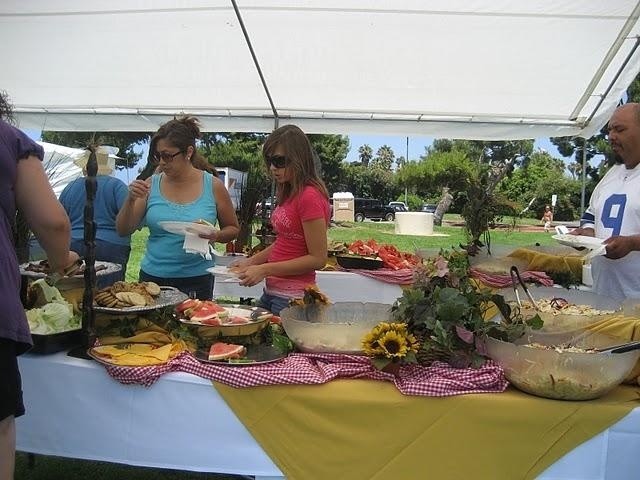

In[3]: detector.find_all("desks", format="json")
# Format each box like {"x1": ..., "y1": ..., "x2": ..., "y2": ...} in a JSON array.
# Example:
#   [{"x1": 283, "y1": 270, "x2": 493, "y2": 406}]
[
  {"x1": 203, "y1": 267, "x2": 590, "y2": 322},
  {"x1": 15, "y1": 340, "x2": 640, "y2": 480}
]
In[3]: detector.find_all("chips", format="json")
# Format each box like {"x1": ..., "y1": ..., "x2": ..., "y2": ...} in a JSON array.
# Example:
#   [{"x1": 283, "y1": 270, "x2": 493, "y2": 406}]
[{"x1": 93, "y1": 343, "x2": 182, "y2": 365}]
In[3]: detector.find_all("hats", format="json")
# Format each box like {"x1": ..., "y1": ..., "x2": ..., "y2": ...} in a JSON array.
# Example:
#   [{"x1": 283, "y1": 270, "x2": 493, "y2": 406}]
[{"x1": 72, "y1": 146, "x2": 114, "y2": 176}]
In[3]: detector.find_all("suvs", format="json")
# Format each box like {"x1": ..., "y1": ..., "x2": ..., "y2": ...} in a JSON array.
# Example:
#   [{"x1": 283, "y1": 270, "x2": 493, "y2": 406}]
[
  {"x1": 354, "y1": 197, "x2": 396, "y2": 224},
  {"x1": 256, "y1": 196, "x2": 278, "y2": 217}
]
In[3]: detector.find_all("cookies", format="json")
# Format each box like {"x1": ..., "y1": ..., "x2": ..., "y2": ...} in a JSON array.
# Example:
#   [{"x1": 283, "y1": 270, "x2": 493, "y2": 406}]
[{"x1": 94, "y1": 280, "x2": 161, "y2": 308}]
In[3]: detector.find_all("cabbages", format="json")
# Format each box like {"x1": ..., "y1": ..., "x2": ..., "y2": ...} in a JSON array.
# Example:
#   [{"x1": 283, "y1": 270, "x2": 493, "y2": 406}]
[{"x1": 25, "y1": 279, "x2": 80, "y2": 334}]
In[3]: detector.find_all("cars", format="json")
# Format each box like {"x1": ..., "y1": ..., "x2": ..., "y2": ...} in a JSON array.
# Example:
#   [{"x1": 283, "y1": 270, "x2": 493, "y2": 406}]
[
  {"x1": 329, "y1": 197, "x2": 334, "y2": 217},
  {"x1": 390, "y1": 201, "x2": 437, "y2": 214}
]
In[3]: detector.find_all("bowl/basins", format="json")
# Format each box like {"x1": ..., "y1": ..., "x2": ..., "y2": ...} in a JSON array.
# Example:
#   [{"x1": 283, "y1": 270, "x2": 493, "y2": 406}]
[
  {"x1": 482, "y1": 326, "x2": 640, "y2": 401},
  {"x1": 414, "y1": 248, "x2": 448, "y2": 259},
  {"x1": 23, "y1": 287, "x2": 275, "y2": 351},
  {"x1": 336, "y1": 254, "x2": 383, "y2": 270},
  {"x1": 501, "y1": 301, "x2": 624, "y2": 326},
  {"x1": 280, "y1": 300, "x2": 397, "y2": 355}
]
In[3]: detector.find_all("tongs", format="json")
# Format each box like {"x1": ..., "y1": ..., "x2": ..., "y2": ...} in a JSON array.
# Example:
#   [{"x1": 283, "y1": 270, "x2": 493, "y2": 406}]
[
  {"x1": 585, "y1": 338, "x2": 639, "y2": 353},
  {"x1": 509, "y1": 263, "x2": 540, "y2": 309}
]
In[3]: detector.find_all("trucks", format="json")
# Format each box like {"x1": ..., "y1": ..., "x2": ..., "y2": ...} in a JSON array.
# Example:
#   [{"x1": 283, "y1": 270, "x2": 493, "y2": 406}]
[{"x1": 213, "y1": 165, "x2": 248, "y2": 213}]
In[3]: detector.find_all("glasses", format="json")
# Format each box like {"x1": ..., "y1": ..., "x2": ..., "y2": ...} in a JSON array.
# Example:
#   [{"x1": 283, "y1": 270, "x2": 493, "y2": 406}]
[
  {"x1": 151, "y1": 148, "x2": 185, "y2": 164},
  {"x1": 263, "y1": 154, "x2": 297, "y2": 168}
]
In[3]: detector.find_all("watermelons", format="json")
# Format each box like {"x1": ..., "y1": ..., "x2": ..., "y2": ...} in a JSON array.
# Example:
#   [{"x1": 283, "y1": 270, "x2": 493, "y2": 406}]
[
  {"x1": 177, "y1": 298, "x2": 248, "y2": 326},
  {"x1": 271, "y1": 316, "x2": 282, "y2": 325},
  {"x1": 209, "y1": 341, "x2": 247, "y2": 361}
]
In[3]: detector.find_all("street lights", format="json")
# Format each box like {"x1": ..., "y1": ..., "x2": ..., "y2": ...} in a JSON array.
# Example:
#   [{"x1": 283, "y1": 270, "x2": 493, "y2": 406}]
[{"x1": 106, "y1": 153, "x2": 131, "y2": 185}]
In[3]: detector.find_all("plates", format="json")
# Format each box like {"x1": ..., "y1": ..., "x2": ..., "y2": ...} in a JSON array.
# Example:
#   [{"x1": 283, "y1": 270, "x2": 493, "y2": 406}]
[
  {"x1": 204, "y1": 266, "x2": 245, "y2": 276},
  {"x1": 551, "y1": 232, "x2": 603, "y2": 248},
  {"x1": 157, "y1": 221, "x2": 215, "y2": 238}
]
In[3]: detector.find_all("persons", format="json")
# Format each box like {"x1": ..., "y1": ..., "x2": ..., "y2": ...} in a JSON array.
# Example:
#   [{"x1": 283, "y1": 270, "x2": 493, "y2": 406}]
[
  {"x1": 566, "y1": 102, "x2": 640, "y2": 303},
  {"x1": 59, "y1": 148, "x2": 142, "y2": 288},
  {"x1": 541, "y1": 205, "x2": 553, "y2": 232},
  {"x1": 1, "y1": 92, "x2": 82, "y2": 479},
  {"x1": 224, "y1": 123, "x2": 330, "y2": 320},
  {"x1": 115, "y1": 114, "x2": 240, "y2": 300}
]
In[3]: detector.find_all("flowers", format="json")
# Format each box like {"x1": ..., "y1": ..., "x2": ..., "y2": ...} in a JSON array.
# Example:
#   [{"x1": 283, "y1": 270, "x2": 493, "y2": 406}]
[{"x1": 358, "y1": 252, "x2": 493, "y2": 364}]
